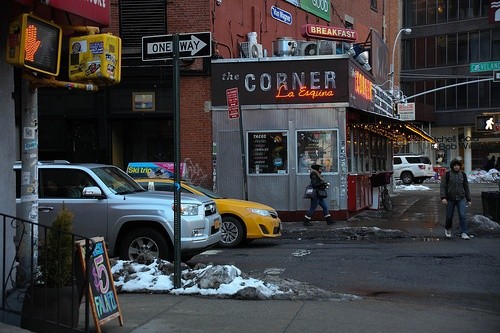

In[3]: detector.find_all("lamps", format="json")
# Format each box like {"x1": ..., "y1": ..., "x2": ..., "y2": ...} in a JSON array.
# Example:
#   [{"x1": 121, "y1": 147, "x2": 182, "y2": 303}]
[
  {"x1": 357, "y1": 50, "x2": 371, "y2": 71},
  {"x1": 378, "y1": 80, "x2": 407, "y2": 107}
]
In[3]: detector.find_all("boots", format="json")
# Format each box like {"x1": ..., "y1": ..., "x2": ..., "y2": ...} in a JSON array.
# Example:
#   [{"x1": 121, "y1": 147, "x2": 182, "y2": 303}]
[
  {"x1": 324, "y1": 214, "x2": 336, "y2": 225},
  {"x1": 303, "y1": 215, "x2": 313, "y2": 226}
]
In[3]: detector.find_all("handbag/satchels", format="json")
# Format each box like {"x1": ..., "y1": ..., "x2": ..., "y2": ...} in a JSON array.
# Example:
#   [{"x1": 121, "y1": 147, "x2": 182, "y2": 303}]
[
  {"x1": 303, "y1": 185, "x2": 314, "y2": 199},
  {"x1": 316, "y1": 190, "x2": 327, "y2": 198}
]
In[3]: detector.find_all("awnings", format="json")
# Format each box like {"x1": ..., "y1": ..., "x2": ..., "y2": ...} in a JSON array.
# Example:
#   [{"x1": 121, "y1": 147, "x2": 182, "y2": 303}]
[{"x1": 350, "y1": 107, "x2": 436, "y2": 145}]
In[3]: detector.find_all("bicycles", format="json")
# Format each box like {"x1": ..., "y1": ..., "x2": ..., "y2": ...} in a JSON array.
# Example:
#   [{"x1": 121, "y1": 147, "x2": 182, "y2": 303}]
[{"x1": 380, "y1": 171, "x2": 394, "y2": 211}]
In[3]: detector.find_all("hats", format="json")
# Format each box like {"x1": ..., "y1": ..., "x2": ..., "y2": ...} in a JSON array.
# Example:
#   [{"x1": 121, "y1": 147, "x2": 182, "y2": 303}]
[{"x1": 311, "y1": 164, "x2": 323, "y2": 170}]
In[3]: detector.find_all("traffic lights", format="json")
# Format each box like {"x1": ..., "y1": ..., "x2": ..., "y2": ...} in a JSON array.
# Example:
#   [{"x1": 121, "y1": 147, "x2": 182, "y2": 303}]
[
  {"x1": 6, "y1": 12, "x2": 62, "y2": 77},
  {"x1": 475, "y1": 114, "x2": 496, "y2": 132},
  {"x1": 432, "y1": 143, "x2": 439, "y2": 148},
  {"x1": 493, "y1": 69, "x2": 500, "y2": 82}
]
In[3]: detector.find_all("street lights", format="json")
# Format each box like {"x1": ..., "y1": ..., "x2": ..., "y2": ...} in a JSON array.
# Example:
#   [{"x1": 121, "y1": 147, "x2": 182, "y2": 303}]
[{"x1": 392, "y1": 29, "x2": 411, "y2": 92}]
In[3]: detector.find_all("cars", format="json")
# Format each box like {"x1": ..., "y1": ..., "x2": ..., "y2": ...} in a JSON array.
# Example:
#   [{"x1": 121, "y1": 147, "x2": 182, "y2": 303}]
[
  {"x1": 432, "y1": 162, "x2": 446, "y2": 178},
  {"x1": 112, "y1": 162, "x2": 278, "y2": 247}
]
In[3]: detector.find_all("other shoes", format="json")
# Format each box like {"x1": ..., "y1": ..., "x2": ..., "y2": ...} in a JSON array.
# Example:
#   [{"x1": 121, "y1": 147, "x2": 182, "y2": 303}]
[
  {"x1": 462, "y1": 232, "x2": 469, "y2": 240},
  {"x1": 444, "y1": 228, "x2": 451, "y2": 238}
]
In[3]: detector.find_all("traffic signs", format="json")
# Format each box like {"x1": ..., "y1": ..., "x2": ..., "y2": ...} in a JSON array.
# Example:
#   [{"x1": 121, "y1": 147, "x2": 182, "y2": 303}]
[{"x1": 141, "y1": 31, "x2": 212, "y2": 61}]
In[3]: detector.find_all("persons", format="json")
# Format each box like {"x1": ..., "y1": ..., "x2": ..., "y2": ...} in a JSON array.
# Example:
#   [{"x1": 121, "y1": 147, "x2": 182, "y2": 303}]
[
  {"x1": 302, "y1": 162, "x2": 336, "y2": 227},
  {"x1": 440, "y1": 158, "x2": 472, "y2": 240}
]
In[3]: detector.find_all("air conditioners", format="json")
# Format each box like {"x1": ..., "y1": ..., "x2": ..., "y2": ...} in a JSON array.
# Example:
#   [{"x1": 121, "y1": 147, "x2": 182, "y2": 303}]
[{"x1": 240, "y1": 42, "x2": 264, "y2": 58}]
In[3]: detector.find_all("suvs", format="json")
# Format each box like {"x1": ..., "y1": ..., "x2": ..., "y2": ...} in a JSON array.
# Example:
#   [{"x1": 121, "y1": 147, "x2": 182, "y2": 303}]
[
  {"x1": 392, "y1": 153, "x2": 436, "y2": 184},
  {"x1": 12, "y1": 160, "x2": 222, "y2": 264}
]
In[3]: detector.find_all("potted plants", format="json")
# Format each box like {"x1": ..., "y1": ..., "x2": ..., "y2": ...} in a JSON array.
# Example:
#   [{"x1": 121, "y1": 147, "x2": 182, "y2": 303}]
[{"x1": 21, "y1": 200, "x2": 85, "y2": 333}]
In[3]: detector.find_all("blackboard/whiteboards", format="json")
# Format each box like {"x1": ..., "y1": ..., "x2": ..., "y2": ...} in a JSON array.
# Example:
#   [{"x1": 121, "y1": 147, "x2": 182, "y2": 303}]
[{"x1": 76, "y1": 237, "x2": 121, "y2": 325}]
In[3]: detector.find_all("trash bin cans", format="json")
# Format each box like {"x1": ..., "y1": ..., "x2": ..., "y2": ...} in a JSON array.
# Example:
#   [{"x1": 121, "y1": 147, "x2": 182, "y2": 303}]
[{"x1": 482, "y1": 191, "x2": 500, "y2": 225}]
